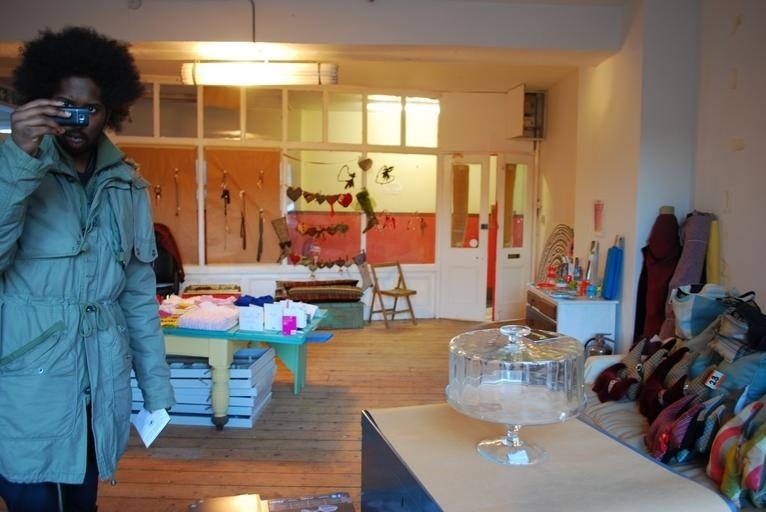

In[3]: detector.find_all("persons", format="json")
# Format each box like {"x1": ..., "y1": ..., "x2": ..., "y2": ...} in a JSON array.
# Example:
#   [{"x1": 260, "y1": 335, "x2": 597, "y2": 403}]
[{"x1": 0, "y1": 24, "x2": 176, "y2": 511}]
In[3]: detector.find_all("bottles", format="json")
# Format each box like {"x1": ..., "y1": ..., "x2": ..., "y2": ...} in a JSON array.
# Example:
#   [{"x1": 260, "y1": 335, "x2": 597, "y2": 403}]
[
  {"x1": 601, "y1": 234, "x2": 623, "y2": 299},
  {"x1": 585, "y1": 240, "x2": 599, "y2": 280},
  {"x1": 573, "y1": 266, "x2": 584, "y2": 281}
]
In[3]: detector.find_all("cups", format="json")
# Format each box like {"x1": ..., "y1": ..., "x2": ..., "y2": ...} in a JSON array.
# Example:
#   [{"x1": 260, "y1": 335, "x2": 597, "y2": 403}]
[
  {"x1": 580, "y1": 281, "x2": 591, "y2": 296},
  {"x1": 586, "y1": 285, "x2": 597, "y2": 298},
  {"x1": 282, "y1": 308, "x2": 296, "y2": 336},
  {"x1": 565, "y1": 275, "x2": 572, "y2": 283}
]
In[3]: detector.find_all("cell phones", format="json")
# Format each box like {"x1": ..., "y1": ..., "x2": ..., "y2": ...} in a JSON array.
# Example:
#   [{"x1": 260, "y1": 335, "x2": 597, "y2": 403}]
[{"x1": 53, "y1": 108, "x2": 90, "y2": 127}]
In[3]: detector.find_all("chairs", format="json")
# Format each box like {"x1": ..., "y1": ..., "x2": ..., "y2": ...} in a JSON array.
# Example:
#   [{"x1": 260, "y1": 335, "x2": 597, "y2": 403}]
[
  {"x1": 366, "y1": 260, "x2": 418, "y2": 328},
  {"x1": 151, "y1": 222, "x2": 184, "y2": 297}
]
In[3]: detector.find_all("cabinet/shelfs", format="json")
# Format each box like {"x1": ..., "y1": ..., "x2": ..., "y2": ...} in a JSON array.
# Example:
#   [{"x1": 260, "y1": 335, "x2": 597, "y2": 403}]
[
  {"x1": 524, "y1": 279, "x2": 620, "y2": 354},
  {"x1": 504, "y1": 85, "x2": 546, "y2": 142},
  {"x1": 361, "y1": 401, "x2": 741, "y2": 512}
]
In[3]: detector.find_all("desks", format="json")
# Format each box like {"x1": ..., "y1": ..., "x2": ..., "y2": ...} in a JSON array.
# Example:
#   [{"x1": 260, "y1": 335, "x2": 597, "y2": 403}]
[{"x1": 161, "y1": 307, "x2": 336, "y2": 432}]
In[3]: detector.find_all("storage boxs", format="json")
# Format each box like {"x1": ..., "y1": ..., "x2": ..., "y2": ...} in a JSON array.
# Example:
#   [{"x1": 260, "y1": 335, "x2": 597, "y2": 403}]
[{"x1": 309, "y1": 301, "x2": 365, "y2": 330}]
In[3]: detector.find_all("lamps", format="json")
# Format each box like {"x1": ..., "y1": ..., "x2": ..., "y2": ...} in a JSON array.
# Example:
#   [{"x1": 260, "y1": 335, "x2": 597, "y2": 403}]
[{"x1": 176, "y1": 54, "x2": 338, "y2": 89}]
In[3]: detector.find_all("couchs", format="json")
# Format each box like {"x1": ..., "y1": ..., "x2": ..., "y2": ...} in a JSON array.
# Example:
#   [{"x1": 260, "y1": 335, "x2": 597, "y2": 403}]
[{"x1": 580, "y1": 351, "x2": 739, "y2": 512}]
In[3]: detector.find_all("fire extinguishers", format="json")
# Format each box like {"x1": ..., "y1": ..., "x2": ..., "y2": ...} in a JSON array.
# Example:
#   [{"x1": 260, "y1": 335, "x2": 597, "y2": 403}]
[{"x1": 584, "y1": 333, "x2": 616, "y2": 357}]
